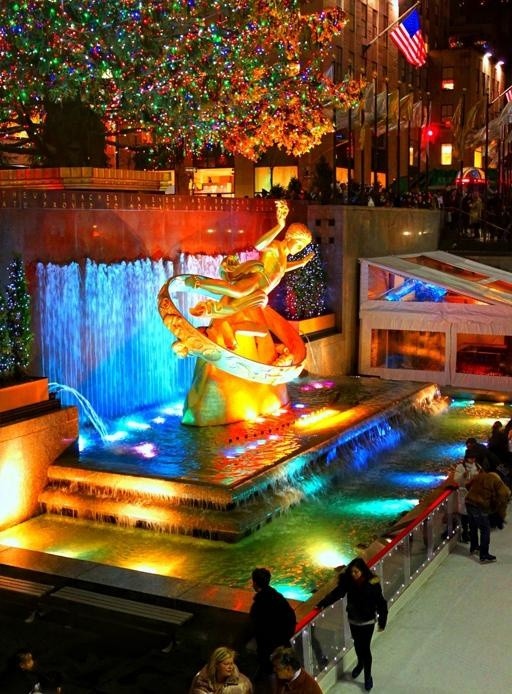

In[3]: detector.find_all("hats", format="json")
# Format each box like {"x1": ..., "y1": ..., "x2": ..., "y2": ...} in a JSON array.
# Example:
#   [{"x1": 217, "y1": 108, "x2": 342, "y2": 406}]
[{"x1": 465, "y1": 449, "x2": 476, "y2": 458}]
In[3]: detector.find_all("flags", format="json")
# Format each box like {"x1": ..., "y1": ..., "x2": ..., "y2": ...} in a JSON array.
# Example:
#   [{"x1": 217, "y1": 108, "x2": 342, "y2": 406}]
[{"x1": 389, "y1": 9, "x2": 427, "y2": 70}]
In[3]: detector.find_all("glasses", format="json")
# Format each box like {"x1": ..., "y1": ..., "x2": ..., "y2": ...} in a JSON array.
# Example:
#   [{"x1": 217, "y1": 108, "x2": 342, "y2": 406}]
[{"x1": 274, "y1": 667, "x2": 284, "y2": 673}]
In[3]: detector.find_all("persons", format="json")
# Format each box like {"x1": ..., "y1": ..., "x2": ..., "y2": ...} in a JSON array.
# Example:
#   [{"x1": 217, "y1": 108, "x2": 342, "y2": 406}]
[
  {"x1": 340, "y1": 183, "x2": 349, "y2": 203},
  {"x1": 269, "y1": 646, "x2": 323, "y2": 694},
  {"x1": 184, "y1": 199, "x2": 315, "y2": 318},
  {"x1": 454, "y1": 419, "x2": 512, "y2": 563},
  {"x1": 9, "y1": 651, "x2": 49, "y2": 694},
  {"x1": 248, "y1": 568, "x2": 296, "y2": 694},
  {"x1": 189, "y1": 646, "x2": 254, "y2": 694},
  {"x1": 444, "y1": 185, "x2": 483, "y2": 237},
  {"x1": 313, "y1": 557, "x2": 388, "y2": 690}
]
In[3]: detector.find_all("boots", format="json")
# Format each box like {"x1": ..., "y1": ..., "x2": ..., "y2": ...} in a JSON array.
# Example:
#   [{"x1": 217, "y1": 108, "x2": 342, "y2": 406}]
[
  {"x1": 365, "y1": 678, "x2": 373, "y2": 690},
  {"x1": 462, "y1": 530, "x2": 469, "y2": 542},
  {"x1": 352, "y1": 665, "x2": 362, "y2": 678},
  {"x1": 470, "y1": 542, "x2": 496, "y2": 562}
]
